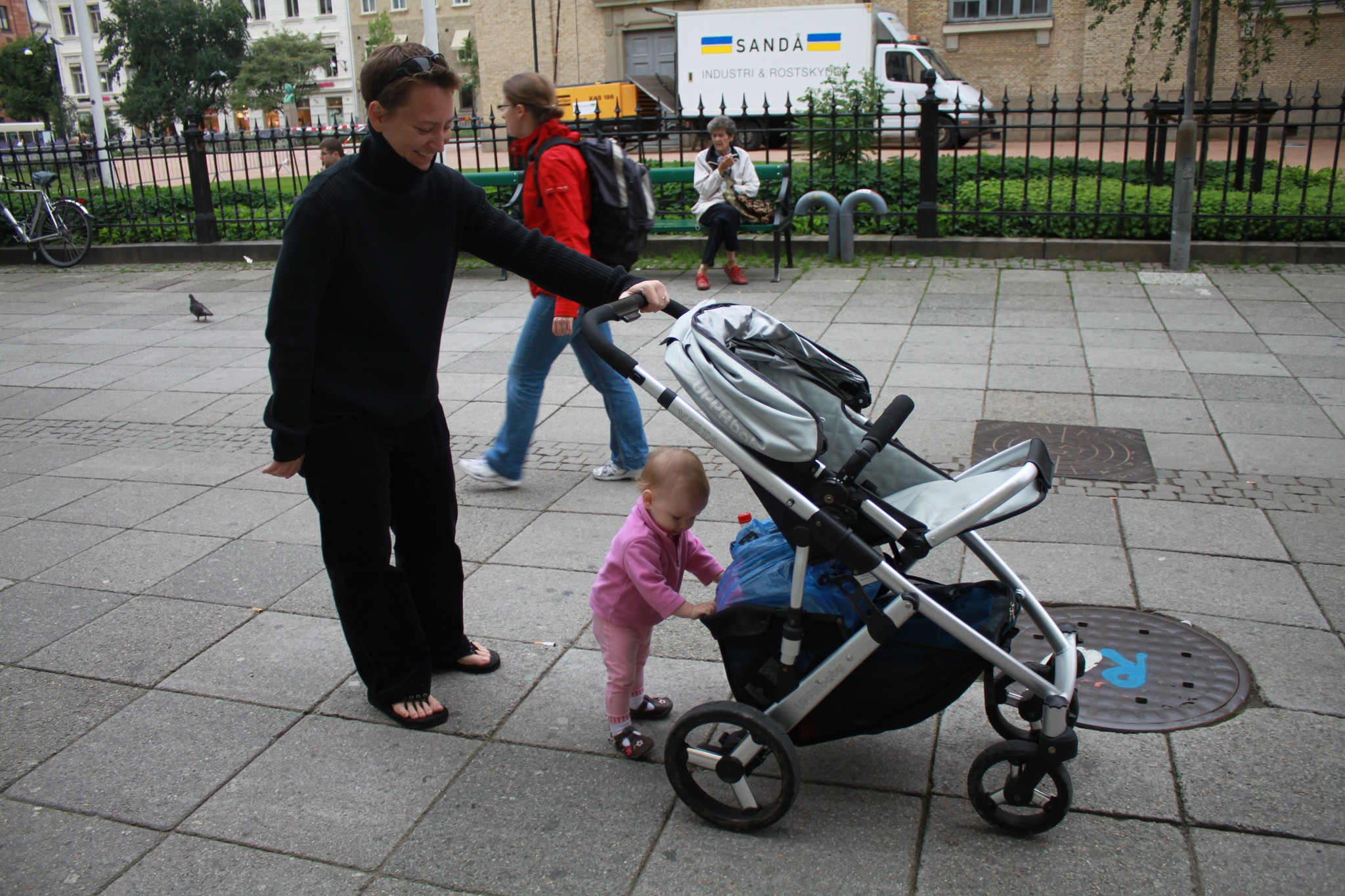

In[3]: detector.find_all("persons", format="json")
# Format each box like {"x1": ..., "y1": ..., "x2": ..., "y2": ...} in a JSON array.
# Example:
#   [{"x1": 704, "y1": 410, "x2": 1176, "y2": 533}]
[
  {"x1": 459, "y1": 74, "x2": 648, "y2": 487},
  {"x1": 204, "y1": 128, "x2": 209, "y2": 135},
  {"x1": 17, "y1": 139, "x2": 24, "y2": 147},
  {"x1": 318, "y1": 137, "x2": 346, "y2": 168},
  {"x1": 283, "y1": 83, "x2": 295, "y2": 103},
  {"x1": 68, "y1": 136, "x2": 79, "y2": 148},
  {"x1": 261, "y1": 44, "x2": 670, "y2": 727},
  {"x1": 76, "y1": 133, "x2": 99, "y2": 176},
  {"x1": 590, "y1": 447, "x2": 727, "y2": 759},
  {"x1": 691, "y1": 115, "x2": 760, "y2": 290}
]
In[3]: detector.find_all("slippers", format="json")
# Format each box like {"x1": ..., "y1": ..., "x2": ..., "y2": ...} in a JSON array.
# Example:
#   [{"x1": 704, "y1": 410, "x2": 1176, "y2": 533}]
[
  {"x1": 444, "y1": 640, "x2": 502, "y2": 673},
  {"x1": 367, "y1": 689, "x2": 449, "y2": 729}
]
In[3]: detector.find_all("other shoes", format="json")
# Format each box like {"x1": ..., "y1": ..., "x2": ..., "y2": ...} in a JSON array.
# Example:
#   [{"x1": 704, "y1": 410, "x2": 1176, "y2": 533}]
[
  {"x1": 630, "y1": 695, "x2": 673, "y2": 719},
  {"x1": 608, "y1": 725, "x2": 655, "y2": 759}
]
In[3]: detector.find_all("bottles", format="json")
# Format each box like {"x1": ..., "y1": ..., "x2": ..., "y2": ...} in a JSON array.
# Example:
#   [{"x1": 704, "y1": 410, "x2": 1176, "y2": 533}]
[{"x1": 738, "y1": 512, "x2": 752, "y2": 529}]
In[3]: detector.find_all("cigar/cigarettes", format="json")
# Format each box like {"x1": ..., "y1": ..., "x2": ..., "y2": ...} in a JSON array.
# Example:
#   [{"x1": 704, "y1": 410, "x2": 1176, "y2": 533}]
[{"x1": 731, "y1": 153, "x2": 738, "y2": 156}]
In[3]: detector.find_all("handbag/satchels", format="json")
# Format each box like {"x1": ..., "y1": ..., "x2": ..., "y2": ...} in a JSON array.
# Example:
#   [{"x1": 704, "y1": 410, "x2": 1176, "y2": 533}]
[
  {"x1": 722, "y1": 190, "x2": 758, "y2": 223},
  {"x1": 739, "y1": 193, "x2": 774, "y2": 223}
]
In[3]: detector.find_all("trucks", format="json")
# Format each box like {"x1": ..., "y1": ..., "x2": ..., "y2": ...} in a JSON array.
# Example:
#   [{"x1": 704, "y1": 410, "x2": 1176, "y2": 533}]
[{"x1": 675, "y1": 2, "x2": 998, "y2": 147}]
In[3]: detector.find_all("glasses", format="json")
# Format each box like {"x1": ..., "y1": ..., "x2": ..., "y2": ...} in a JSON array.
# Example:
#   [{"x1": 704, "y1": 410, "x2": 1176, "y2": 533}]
[
  {"x1": 499, "y1": 103, "x2": 514, "y2": 111},
  {"x1": 372, "y1": 53, "x2": 445, "y2": 101}
]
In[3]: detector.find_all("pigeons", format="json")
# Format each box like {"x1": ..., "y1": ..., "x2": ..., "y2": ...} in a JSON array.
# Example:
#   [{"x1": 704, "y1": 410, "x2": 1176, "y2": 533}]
[{"x1": 189, "y1": 294, "x2": 213, "y2": 322}]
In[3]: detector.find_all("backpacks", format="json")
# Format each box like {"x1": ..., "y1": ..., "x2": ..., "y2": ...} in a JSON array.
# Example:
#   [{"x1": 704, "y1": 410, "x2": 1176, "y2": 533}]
[{"x1": 525, "y1": 135, "x2": 657, "y2": 271}]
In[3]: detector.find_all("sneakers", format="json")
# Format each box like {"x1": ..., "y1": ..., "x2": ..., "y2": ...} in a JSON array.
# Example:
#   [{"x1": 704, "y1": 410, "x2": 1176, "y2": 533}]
[
  {"x1": 592, "y1": 460, "x2": 645, "y2": 480},
  {"x1": 459, "y1": 458, "x2": 522, "y2": 487}
]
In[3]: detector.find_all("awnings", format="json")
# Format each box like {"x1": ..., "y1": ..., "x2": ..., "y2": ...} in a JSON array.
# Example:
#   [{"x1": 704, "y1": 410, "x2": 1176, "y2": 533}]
[
  {"x1": 451, "y1": 30, "x2": 469, "y2": 50},
  {"x1": 389, "y1": 34, "x2": 407, "y2": 43},
  {"x1": 456, "y1": 73, "x2": 474, "y2": 85}
]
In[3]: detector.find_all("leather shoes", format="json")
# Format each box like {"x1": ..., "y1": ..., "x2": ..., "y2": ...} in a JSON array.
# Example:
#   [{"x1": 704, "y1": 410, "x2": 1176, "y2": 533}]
[
  {"x1": 696, "y1": 273, "x2": 710, "y2": 289},
  {"x1": 724, "y1": 262, "x2": 748, "y2": 284}
]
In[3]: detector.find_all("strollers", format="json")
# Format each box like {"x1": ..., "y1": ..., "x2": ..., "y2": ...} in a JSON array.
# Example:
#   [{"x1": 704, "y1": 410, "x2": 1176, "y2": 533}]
[{"x1": 582, "y1": 298, "x2": 1100, "y2": 836}]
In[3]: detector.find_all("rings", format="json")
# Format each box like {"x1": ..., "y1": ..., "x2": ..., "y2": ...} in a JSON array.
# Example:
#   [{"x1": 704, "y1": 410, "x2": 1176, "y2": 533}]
[{"x1": 662, "y1": 296, "x2": 666, "y2": 299}]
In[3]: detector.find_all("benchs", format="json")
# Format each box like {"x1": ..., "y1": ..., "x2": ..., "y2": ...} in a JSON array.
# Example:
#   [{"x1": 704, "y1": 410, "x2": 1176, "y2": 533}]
[{"x1": 463, "y1": 163, "x2": 796, "y2": 284}]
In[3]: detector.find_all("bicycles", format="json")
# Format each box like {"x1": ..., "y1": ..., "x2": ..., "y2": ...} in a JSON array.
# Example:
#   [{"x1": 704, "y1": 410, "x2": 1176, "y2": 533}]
[{"x1": 0, "y1": 170, "x2": 94, "y2": 266}]
